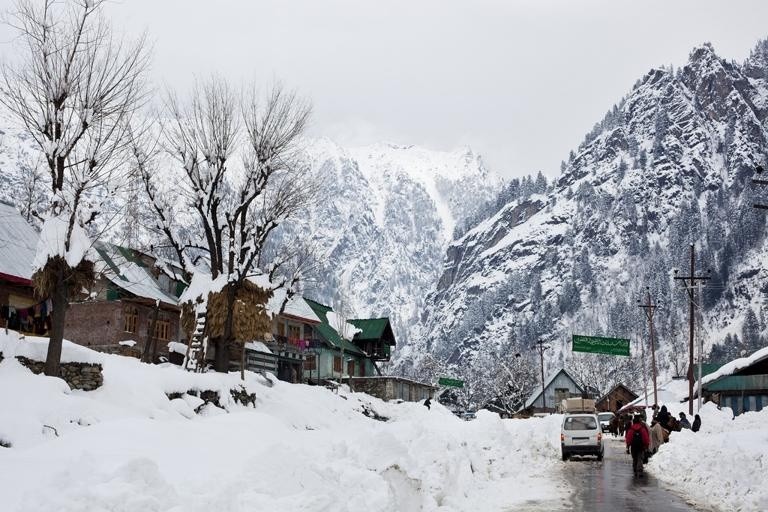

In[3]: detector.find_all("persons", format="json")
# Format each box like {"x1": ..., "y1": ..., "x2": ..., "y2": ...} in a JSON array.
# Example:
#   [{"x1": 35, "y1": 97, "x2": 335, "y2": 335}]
[
  {"x1": 608, "y1": 403, "x2": 702, "y2": 463},
  {"x1": 625, "y1": 415, "x2": 650, "y2": 477}
]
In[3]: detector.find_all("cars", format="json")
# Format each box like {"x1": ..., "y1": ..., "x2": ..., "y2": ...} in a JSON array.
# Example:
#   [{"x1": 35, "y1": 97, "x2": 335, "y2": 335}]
[{"x1": 599, "y1": 411, "x2": 615, "y2": 432}]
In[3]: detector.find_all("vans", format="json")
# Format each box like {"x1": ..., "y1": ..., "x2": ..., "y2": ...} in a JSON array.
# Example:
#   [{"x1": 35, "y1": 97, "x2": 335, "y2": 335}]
[{"x1": 561, "y1": 414, "x2": 604, "y2": 461}]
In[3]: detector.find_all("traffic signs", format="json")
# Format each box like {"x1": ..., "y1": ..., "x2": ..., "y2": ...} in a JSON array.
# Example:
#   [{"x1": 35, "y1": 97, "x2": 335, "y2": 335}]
[
  {"x1": 573, "y1": 335, "x2": 630, "y2": 355},
  {"x1": 438, "y1": 377, "x2": 463, "y2": 388}
]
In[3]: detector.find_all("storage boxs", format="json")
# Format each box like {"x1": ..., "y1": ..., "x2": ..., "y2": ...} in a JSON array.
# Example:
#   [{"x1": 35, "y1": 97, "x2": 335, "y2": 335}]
[
  {"x1": 582, "y1": 399, "x2": 595, "y2": 412},
  {"x1": 566, "y1": 397, "x2": 582, "y2": 412}
]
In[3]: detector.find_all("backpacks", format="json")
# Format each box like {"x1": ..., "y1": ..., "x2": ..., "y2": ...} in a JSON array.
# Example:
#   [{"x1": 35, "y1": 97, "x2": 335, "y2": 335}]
[{"x1": 630, "y1": 427, "x2": 644, "y2": 454}]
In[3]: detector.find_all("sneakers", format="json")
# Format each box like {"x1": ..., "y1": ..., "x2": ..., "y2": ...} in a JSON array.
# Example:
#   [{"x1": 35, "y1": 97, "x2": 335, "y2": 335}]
[{"x1": 632, "y1": 472, "x2": 646, "y2": 479}]
